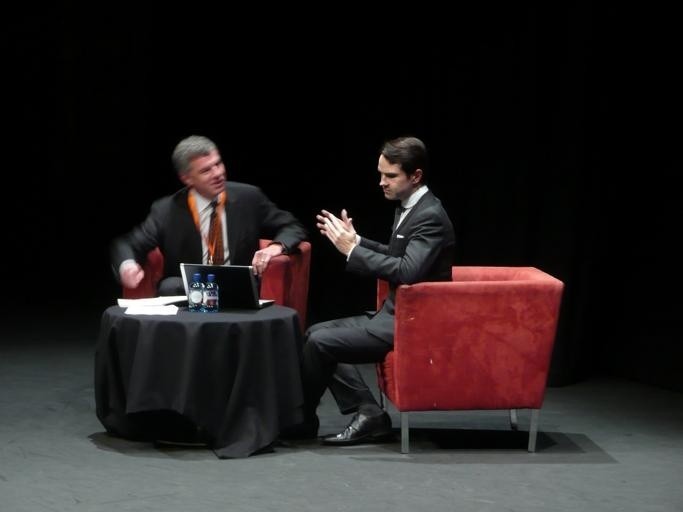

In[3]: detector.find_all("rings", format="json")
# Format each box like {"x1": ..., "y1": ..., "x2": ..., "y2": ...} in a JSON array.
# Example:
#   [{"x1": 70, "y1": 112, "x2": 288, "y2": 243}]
[{"x1": 261, "y1": 259, "x2": 265, "y2": 261}]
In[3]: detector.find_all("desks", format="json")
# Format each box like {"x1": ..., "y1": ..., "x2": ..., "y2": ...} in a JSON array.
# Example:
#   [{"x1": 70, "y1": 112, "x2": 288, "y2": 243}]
[{"x1": 103, "y1": 304, "x2": 299, "y2": 452}]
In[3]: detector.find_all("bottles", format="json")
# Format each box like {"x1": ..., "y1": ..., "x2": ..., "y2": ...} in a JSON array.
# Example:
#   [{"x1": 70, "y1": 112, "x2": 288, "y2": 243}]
[
  {"x1": 188, "y1": 273, "x2": 206, "y2": 313},
  {"x1": 202, "y1": 274, "x2": 219, "y2": 314}
]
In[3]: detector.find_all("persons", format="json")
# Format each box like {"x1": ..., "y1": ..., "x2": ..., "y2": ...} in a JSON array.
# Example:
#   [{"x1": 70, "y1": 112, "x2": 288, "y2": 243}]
[
  {"x1": 111, "y1": 135, "x2": 310, "y2": 298},
  {"x1": 280, "y1": 135, "x2": 455, "y2": 445}
]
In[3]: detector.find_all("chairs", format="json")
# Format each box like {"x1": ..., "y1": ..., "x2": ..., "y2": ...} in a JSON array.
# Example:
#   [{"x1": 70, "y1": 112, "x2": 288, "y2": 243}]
[
  {"x1": 374, "y1": 262, "x2": 565, "y2": 452},
  {"x1": 118, "y1": 240, "x2": 311, "y2": 331}
]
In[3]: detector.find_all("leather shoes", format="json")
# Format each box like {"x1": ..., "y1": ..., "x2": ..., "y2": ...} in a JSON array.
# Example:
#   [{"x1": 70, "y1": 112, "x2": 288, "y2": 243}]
[{"x1": 321, "y1": 412, "x2": 392, "y2": 444}]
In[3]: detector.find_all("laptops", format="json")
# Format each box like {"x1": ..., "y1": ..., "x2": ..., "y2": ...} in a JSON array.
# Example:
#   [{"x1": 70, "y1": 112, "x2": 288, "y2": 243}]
[{"x1": 179, "y1": 263, "x2": 275, "y2": 310}]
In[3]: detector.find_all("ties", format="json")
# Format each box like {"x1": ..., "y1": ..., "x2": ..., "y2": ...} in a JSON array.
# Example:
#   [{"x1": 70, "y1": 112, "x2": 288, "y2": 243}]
[{"x1": 208, "y1": 202, "x2": 224, "y2": 265}]
